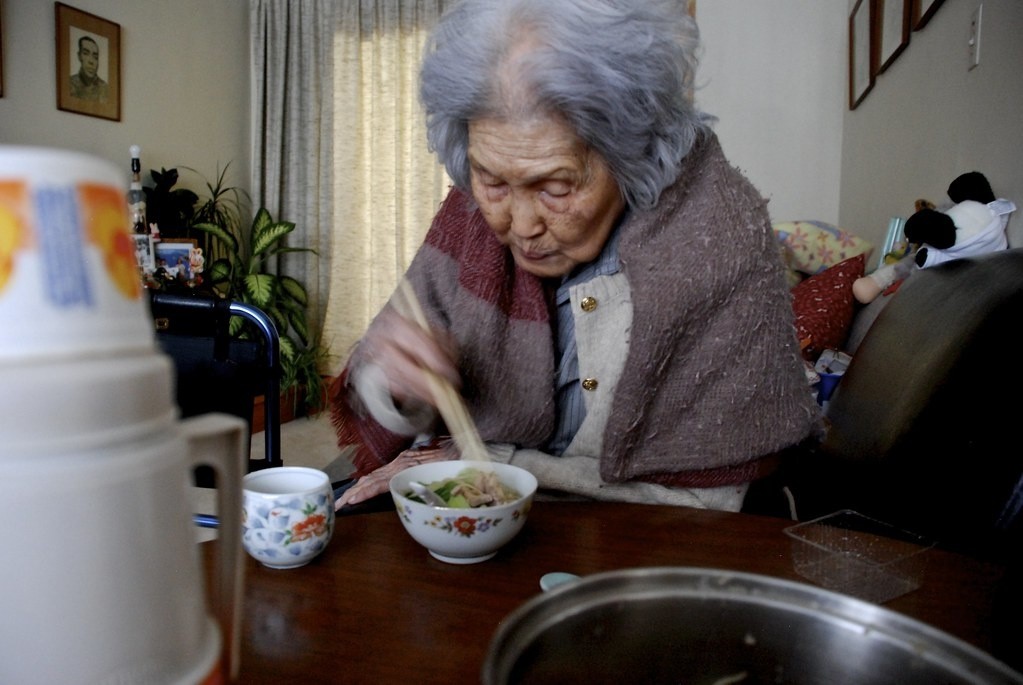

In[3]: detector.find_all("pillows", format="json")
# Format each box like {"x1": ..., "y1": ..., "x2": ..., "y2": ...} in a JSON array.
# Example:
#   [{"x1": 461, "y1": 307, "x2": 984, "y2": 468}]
[
  {"x1": 771, "y1": 220, "x2": 874, "y2": 277},
  {"x1": 790, "y1": 252, "x2": 866, "y2": 362}
]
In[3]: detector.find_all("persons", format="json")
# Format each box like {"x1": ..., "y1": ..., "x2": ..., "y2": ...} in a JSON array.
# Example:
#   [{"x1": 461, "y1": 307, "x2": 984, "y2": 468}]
[
  {"x1": 67, "y1": 35, "x2": 109, "y2": 105},
  {"x1": 134, "y1": 241, "x2": 186, "y2": 279},
  {"x1": 325, "y1": 0, "x2": 817, "y2": 511}
]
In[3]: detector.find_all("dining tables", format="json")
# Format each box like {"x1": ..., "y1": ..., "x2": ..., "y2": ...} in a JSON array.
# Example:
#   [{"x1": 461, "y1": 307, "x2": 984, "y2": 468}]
[{"x1": 195, "y1": 500, "x2": 1022, "y2": 685}]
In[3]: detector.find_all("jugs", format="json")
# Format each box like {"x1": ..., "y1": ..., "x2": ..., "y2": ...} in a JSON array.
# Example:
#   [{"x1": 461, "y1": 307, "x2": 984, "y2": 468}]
[{"x1": 0, "y1": 353, "x2": 243, "y2": 683}]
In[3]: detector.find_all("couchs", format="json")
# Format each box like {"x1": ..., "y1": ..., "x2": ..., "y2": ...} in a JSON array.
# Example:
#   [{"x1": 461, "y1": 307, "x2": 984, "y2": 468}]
[{"x1": 823, "y1": 246, "x2": 1023, "y2": 571}]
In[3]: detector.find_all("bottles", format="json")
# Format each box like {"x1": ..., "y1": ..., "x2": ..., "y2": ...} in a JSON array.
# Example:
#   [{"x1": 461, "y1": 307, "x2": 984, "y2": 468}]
[{"x1": 126, "y1": 142, "x2": 156, "y2": 293}]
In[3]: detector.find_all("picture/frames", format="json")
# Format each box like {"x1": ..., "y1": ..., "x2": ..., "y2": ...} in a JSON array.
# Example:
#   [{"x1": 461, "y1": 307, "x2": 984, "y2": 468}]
[
  {"x1": 53, "y1": 0, "x2": 122, "y2": 123},
  {"x1": 913, "y1": 0, "x2": 943, "y2": 32},
  {"x1": 848, "y1": 0, "x2": 872, "y2": 110},
  {"x1": 873, "y1": 1, "x2": 911, "y2": 78},
  {"x1": 154, "y1": 239, "x2": 204, "y2": 285}
]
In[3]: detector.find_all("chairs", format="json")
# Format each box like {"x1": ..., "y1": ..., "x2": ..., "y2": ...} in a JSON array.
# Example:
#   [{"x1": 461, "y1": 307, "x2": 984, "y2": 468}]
[{"x1": 149, "y1": 290, "x2": 280, "y2": 487}]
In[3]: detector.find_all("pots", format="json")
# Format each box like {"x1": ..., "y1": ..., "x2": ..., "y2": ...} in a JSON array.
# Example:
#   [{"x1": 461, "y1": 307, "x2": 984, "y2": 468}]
[{"x1": 486, "y1": 564, "x2": 1022, "y2": 685}]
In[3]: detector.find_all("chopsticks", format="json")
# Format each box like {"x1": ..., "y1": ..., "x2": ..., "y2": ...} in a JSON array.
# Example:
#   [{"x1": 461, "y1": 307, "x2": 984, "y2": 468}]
[{"x1": 389, "y1": 274, "x2": 490, "y2": 476}]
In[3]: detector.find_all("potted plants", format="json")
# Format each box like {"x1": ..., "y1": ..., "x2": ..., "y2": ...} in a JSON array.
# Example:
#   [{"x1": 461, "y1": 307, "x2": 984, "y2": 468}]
[{"x1": 146, "y1": 165, "x2": 325, "y2": 434}]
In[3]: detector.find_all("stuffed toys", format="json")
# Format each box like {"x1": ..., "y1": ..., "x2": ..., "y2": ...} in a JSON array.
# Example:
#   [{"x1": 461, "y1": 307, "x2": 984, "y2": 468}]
[{"x1": 903, "y1": 169, "x2": 1017, "y2": 270}]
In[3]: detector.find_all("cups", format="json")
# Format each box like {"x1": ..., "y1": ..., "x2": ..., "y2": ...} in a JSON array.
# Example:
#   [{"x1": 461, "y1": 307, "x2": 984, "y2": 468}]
[
  {"x1": 241, "y1": 466, "x2": 335, "y2": 569},
  {"x1": 1, "y1": 141, "x2": 158, "y2": 360}
]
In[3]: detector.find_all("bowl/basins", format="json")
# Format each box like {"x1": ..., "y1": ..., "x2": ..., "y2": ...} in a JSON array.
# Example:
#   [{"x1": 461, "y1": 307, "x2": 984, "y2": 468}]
[{"x1": 388, "y1": 457, "x2": 538, "y2": 565}]
